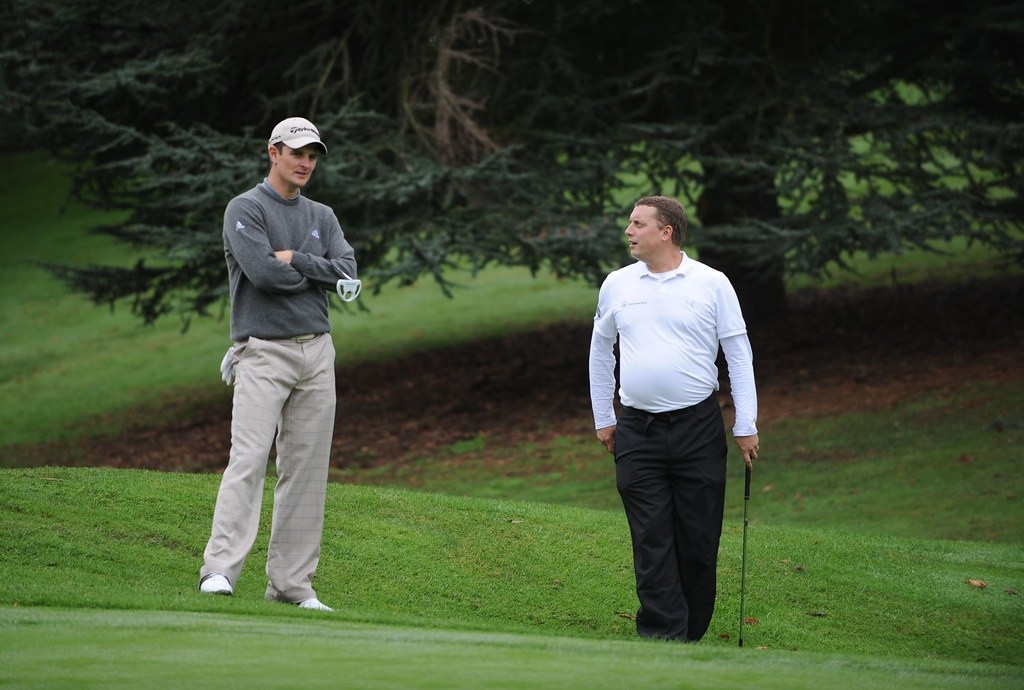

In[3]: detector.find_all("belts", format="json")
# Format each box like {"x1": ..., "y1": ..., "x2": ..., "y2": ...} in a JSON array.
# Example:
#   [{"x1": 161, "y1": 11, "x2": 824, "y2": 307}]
[{"x1": 255, "y1": 331, "x2": 329, "y2": 343}]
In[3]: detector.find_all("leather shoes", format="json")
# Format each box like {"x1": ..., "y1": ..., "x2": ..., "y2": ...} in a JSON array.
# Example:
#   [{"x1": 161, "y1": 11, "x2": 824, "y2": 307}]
[
  {"x1": 200, "y1": 574, "x2": 233, "y2": 596},
  {"x1": 294, "y1": 597, "x2": 337, "y2": 612}
]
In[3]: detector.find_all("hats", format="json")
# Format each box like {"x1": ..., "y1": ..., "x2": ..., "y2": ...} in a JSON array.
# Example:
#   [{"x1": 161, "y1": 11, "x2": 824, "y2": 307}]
[{"x1": 267, "y1": 116, "x2": 328, "y2": 156}]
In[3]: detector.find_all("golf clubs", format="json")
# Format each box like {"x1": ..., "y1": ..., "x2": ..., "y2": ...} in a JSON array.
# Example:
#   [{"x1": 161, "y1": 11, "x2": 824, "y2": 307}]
[{"x1": 737, "y1": 455, "x2": 752, "y2": 649}]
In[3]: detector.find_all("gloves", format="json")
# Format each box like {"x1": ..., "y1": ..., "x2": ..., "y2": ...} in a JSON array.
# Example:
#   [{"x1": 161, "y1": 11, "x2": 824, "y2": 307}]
[{"x1": 219, "y1": 346, "x2": 235, "y2": 387}]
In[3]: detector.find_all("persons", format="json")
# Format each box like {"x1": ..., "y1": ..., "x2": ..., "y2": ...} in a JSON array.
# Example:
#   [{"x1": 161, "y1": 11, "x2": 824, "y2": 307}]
[
  {"x1": 589, "y1": 196, "x2": 759, "y2": 643},
  {"x1": 199, "y1": 117, "x2": 357, "y2": 611}
]
